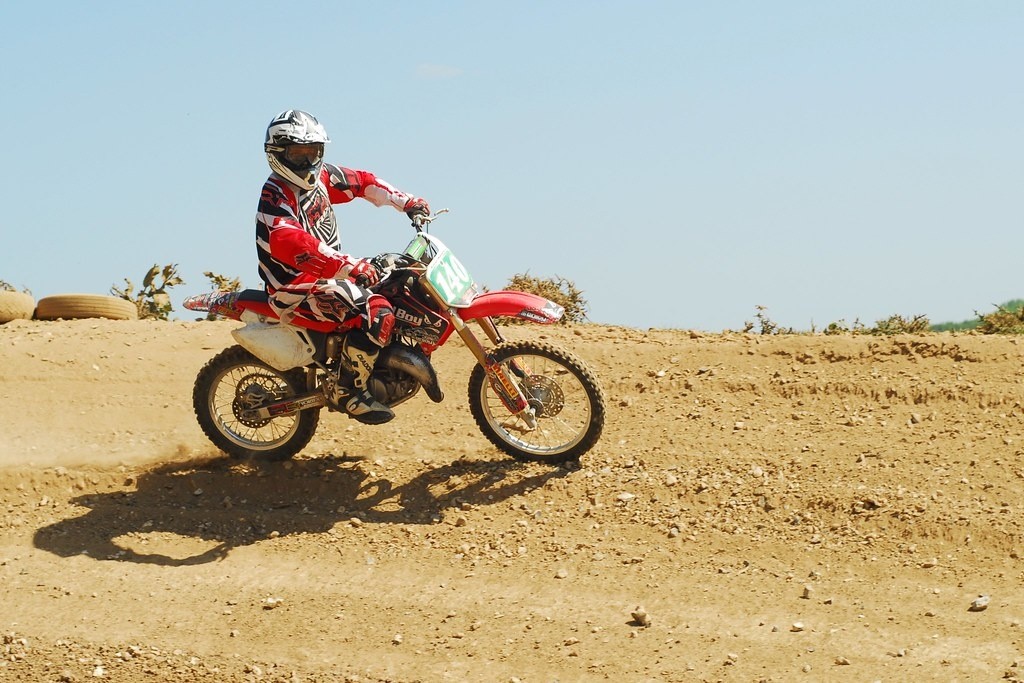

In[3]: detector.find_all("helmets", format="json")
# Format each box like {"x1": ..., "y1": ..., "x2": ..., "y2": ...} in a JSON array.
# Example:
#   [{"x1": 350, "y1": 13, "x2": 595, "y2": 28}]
[{"x1": 264, "y1": 109, "x2": 331, "y2": 191}]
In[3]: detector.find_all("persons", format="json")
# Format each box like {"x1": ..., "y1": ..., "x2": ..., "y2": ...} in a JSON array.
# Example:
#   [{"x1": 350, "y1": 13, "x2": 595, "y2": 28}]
[{"x1": 255, "y1": 109, "x2": 430, "y2": 426}]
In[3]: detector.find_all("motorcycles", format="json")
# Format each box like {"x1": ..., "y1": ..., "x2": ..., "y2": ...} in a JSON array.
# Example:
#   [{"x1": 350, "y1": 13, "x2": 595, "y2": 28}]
[{"x1": 192, "y1": 208, "x2": 607, "y2": 464}]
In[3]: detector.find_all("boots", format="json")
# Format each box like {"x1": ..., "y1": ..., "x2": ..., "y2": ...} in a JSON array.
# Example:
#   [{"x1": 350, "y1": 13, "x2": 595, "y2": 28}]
[{"x1": 320, "y1": 334, "x2": 395, "y2": 425}]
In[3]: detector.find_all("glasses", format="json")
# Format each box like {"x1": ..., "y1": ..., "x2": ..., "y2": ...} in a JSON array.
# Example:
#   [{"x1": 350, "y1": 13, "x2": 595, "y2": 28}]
[{"x1": 285, "y1": 143, "x2": 324, "y2": 167}]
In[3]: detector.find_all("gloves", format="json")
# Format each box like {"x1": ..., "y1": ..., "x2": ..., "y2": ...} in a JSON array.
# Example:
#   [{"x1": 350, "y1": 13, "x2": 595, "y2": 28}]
[
  {"x1": 349, "y1": 257, "x2": 384, "y2": 286},
  {"x1": 404, "y1": 197, "x2": 431, "y2": 222}
]
